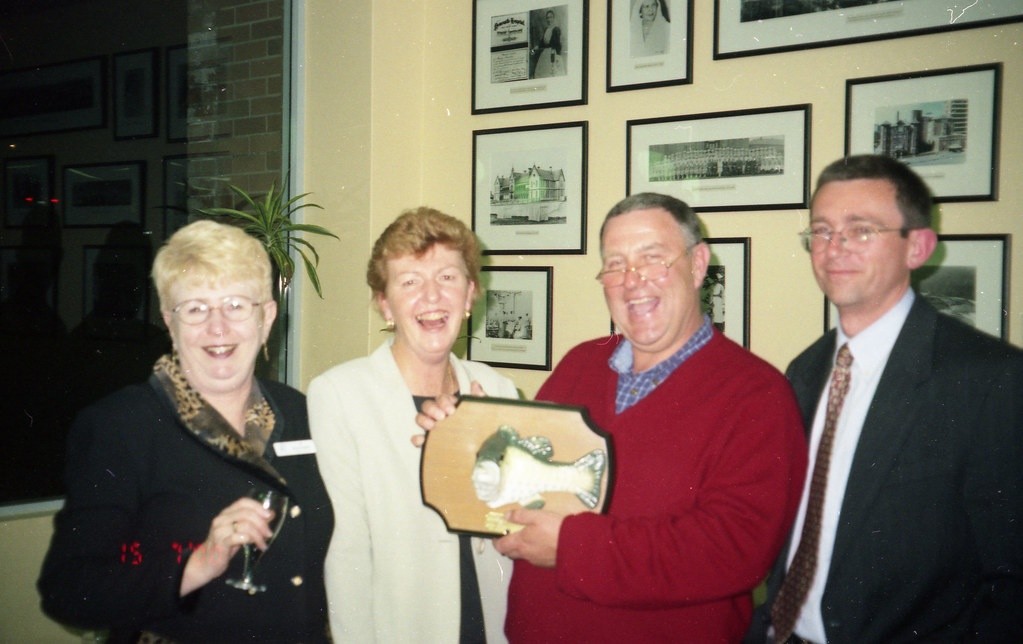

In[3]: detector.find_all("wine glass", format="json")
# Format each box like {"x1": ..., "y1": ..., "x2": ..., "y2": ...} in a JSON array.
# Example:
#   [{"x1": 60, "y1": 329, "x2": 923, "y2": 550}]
[{"x1": 226, "y1": 489, "x2": 287, "y2": 593}]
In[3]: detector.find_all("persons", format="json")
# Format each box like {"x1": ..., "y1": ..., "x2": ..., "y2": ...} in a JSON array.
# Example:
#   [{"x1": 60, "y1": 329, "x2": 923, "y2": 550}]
[
  {"x1": 653, "y1": 148, "x2": 784, "y2": 180},
  {"x1": 531, "y1": 10, "x2": 565, "y2": 78},
  {"x1": 306, "y1": 207, "x2": 519, "y2": 644},
  {"x1": 630, "y1": 0, "x2": 671, "y2": 56},
  {"x1": 739, "y1": 153, "x2": 1023, "y2": 644},
  {"x1": 411, "y1": 192, "x2": 810, "y2": 644},
  {"x1": 37, "y1": 219, "x2": 336, "y2": 644},
  {"x1": 497, "y1": 309, "x2": 529, "y2": 339}
]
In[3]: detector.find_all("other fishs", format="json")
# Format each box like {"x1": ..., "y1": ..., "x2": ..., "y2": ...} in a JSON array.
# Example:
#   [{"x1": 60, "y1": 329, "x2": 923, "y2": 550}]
[{"x1": 471, "y1": 426, "x2": 605, "y2": 512}]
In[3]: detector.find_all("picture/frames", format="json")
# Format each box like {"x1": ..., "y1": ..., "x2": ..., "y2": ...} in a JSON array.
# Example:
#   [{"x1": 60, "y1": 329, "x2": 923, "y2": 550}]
[
  {"x1": 822, "y1": 233, "x2": 1010, "y2": 349},
  {"x1": 472, "y1": 121, "x2": 588, "y2": 254},
  {"x1": 712, "y1": 0, "x2": 1023, "y2": 61},
  {"x1": 606, "y1": 0, "x2": 694, "y2": 91},
  {"x1": 847, "y1": 63, "x2": 1006, "y2": 204},
  {"x1": 606, "y1": 238, "x2": 753, "y2": 353},
  {"x1": 467, "y1": 265, "x2": 553, "y2": 370},
  {"x1": 472, "y1": 0, "x2": 588, "y2": 115},
  {"x1": 625, "y1": 103, "x2": 815, "y2": 208}
]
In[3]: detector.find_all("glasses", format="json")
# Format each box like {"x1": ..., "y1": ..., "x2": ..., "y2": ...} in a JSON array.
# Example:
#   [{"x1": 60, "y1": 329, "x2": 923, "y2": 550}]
[
  {"x1": 799, "y1": 225, "x2": 911, "y2": 253},
  {"x1": 595, "y1": 242, "x2": 697, "y2": 287},
  {"x1": 167, "y1": 295, "x2": 264, "y2": 324}
]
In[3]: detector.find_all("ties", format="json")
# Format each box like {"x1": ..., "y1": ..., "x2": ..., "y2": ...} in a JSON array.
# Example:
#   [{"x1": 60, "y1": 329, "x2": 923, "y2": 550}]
[{"x1": 771, "y1": 344, "x2": 853, "y2": 644}]
